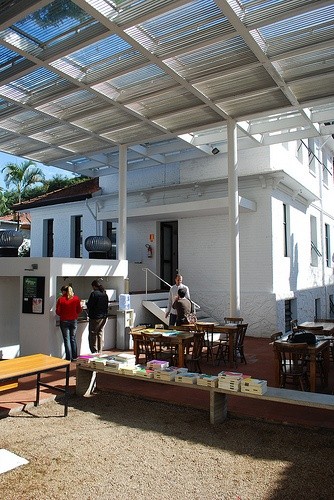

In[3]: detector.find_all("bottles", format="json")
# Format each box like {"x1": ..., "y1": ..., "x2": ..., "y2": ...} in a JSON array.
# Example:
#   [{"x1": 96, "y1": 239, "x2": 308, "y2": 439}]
[{"x1": 119, "y1": 293, "x2": 131, "y2": 310}]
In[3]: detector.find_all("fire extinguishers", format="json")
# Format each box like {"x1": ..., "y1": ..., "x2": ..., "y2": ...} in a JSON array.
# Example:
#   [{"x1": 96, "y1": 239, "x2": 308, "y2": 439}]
[{"x1": 148, "y1": 245, "x2": 152, "y2": 257}]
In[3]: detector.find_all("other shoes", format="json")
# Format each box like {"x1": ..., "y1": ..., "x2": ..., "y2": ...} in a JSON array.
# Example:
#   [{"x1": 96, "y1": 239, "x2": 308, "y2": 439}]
[{"x1": 72, "y1": 356, "x2": 78, "y2": 360}]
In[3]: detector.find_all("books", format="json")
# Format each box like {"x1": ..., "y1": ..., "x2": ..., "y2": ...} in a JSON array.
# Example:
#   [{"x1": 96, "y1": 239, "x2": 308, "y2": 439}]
[
  {"x1": 241, "y1": 378, "x2": 267, "y2": 395},
  {"x1": 76, "y1": 353, "x2": 141, "y2": 376},
  {"x1": 175, "y1": 373, "x2": 198, "y2": 384},
  {"x1": 217, "y1": 371, "x2": 251, "y2": 392},
  {"x1": 154, "y1": 366, "x2": 188, "y2": 381},
  {"x1": 135, "y1": 360, "x2": 169, "y2": 379},
  {"x1": 197, "y1": 374, "x2": 218, "y2": 387}
]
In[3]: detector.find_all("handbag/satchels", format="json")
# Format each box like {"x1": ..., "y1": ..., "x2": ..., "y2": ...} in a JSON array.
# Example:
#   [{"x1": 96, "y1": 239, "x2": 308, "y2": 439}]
[{"x1": 183, "y1": 312, "x2": 197, "y2": 324}]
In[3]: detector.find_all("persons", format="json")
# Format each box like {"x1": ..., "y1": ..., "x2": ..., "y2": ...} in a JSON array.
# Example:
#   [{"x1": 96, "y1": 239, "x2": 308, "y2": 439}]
[
  {"x1": 172, "y1": 288, "x2": 194, "y2": 326},
  {"x1": 165, "y1": 274, "x2": 190, "y2": 331},
  {"x1": 86, "y1": 280, "x2": 109, "y2": 353},
  {"x1": 56, "y1": 286, "x2": 82, "y2": 360}
]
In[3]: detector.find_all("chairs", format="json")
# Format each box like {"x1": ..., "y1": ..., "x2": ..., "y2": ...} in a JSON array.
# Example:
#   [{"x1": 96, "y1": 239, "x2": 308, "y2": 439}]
[
  {"x1": 128, "y1": 317, "x2": 248, "y2": 373},
  {"x1": 268, "y1": 319, "x2": 334, "y2": 393}
]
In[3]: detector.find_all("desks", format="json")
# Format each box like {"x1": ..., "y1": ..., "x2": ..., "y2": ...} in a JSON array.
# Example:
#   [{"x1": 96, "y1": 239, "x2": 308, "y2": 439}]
[
  {"x1": 0, "y1": 353, "x2": 71, "y2": 417},
  {"x1": 181, "y1": 323, "x2": 244, "y2": 367},
  {"x1": 293, "y1": 321, "x2": 334, "y2": 336},
  {"x1": 268, "y1": 332, "x2": 330, "y2": 393},
  {"x1": 132, "y1": 328, "x2": 194, "y2": 368}
]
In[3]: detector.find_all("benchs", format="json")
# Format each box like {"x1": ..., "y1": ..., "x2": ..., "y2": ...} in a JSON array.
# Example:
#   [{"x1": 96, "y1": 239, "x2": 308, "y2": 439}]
[{"x1": 76, "y1": 360, "x2": 334, "y2": 428}]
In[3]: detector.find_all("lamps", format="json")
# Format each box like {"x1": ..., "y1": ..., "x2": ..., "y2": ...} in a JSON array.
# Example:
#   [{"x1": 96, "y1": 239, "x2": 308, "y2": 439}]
[{"x1": 211, "y1": 145, "x2": 220, "y2": 154}]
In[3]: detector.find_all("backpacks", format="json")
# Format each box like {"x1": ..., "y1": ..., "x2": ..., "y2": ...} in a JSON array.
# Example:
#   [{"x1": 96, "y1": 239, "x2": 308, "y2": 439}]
[{"x1": 286, "y1": 329, "x2": 316, "y2": 345}]
